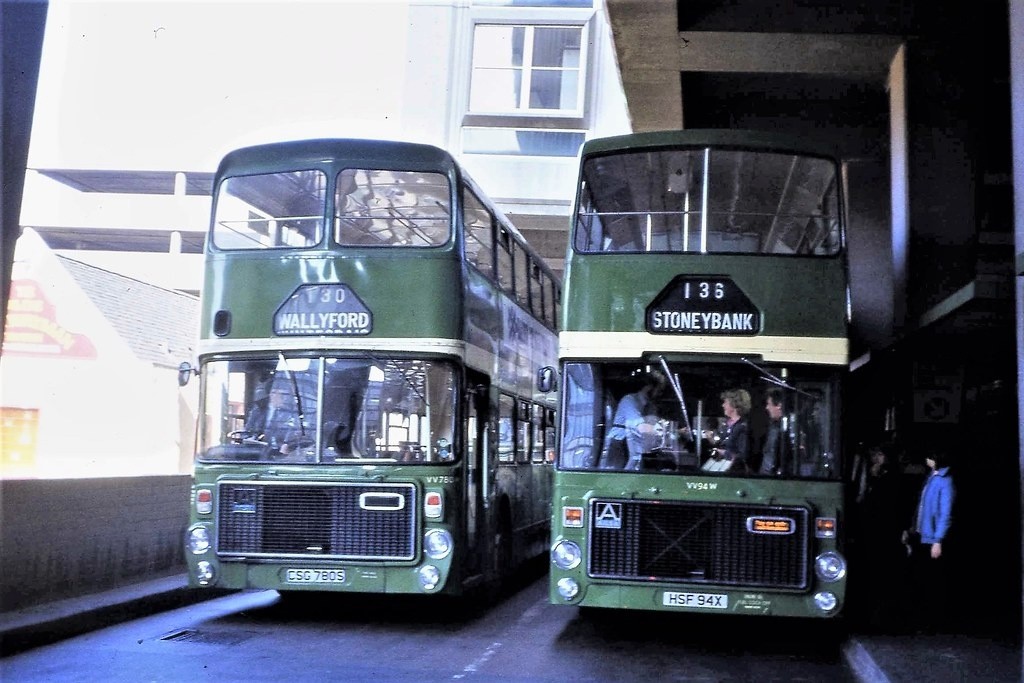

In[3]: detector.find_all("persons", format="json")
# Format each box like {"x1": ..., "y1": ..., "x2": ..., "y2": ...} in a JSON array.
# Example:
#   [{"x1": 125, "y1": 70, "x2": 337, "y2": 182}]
[
  {"x1": 605, "y1": 366, "x2": 691, "y2": 471},
  {"x1": 849, "y1": 439, "x2": 955, "y2": 637},
  {"x1": 238, "y1": 385, "x2": 295, "y2": 455},
  {"x1": 761, "y1": 387, "x2": 808, "y2": 476},
  {"x1": 706, "y1": 389, "x2": 751, "y2": 475},
  {"x1": 810, "y1": 399, "x2": 823, "y2": 426}
]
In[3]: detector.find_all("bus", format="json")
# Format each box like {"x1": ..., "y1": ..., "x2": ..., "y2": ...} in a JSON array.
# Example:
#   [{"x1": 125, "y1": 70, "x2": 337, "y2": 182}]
[
  {"x1": 178, "y1": 138, "x2": 562, "y2": 620},
  {"x1": 536, "y1": 129, "x2": 854, "y2": 620}
]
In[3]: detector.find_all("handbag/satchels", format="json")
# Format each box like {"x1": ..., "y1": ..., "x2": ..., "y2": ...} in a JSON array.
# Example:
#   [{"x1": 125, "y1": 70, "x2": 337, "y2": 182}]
[{"x1": 700, "y1": 457, "x2": 733, "y2": 473}]
